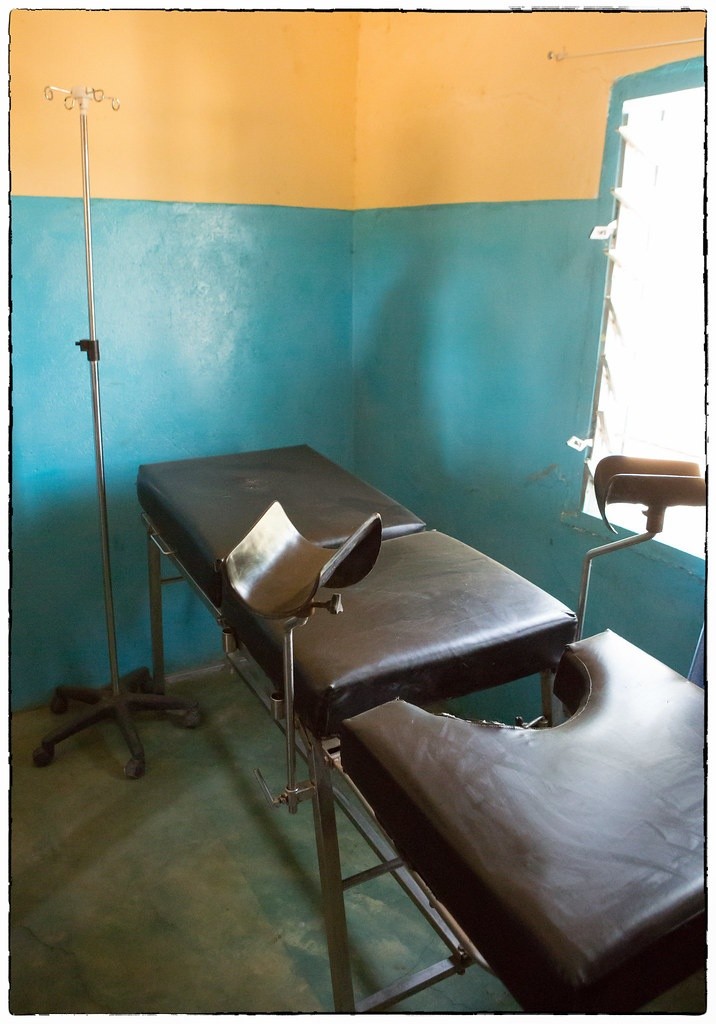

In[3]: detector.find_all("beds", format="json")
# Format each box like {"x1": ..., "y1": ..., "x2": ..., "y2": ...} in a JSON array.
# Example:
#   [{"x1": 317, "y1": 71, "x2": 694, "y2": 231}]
[{"x1": 133, "y1": 445, "x2": 708, "y2": 1014}]
